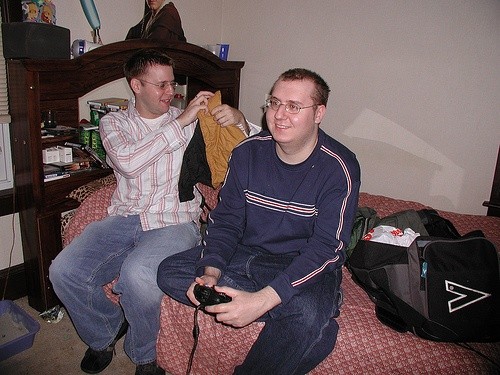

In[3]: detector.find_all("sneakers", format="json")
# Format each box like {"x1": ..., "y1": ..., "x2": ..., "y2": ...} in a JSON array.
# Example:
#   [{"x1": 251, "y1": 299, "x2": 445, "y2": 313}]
[
  {"x1": 135, "y1": 358, "x2": 166, "y2": 375},
  {"x1": 81, "y1": 318, "x2": 127, "y2": 375}
]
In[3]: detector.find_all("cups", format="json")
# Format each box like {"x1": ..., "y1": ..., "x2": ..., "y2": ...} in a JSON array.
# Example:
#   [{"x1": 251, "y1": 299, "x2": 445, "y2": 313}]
[{"x1": 44, "y1": 110, "x2": 57, "y2": 128}]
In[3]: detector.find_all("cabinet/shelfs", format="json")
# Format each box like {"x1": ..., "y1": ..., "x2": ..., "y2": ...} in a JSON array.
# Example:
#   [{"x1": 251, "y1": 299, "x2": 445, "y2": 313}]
[{"x1": 8, "y1": 39, "x2": 247, "y2": 310}]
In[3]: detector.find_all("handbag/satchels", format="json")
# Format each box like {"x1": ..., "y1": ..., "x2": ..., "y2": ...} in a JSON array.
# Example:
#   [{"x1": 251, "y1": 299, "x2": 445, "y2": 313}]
[{"x1": 345, "y1": 209, "x2": 500, "y2": 344}]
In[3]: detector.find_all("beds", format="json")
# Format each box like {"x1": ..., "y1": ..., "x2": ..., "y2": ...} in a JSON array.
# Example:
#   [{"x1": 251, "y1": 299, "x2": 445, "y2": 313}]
[{"x1": 62, "y1": 183, "x2": 500, "y2": 375}]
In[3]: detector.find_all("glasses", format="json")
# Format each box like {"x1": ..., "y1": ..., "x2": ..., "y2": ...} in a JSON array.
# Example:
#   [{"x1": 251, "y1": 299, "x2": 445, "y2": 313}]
[
  {"x1": 136, "y1": 78, "x2": 179, "y2": 90},
  {"x1": 266, "y1": 97, "x2": 322, "y2": 114}
]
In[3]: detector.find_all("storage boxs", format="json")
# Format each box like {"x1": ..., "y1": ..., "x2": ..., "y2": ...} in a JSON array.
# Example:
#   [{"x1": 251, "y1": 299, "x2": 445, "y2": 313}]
[
  {"x1": 43, "y1": 148, "x2": 59, "y2": 164},
  {"x1": 57, "y1": 146, "x2": 73, "y2": 163},
  {"x1": 0, "y1": 300, "x2": 40, "y2": 361}
]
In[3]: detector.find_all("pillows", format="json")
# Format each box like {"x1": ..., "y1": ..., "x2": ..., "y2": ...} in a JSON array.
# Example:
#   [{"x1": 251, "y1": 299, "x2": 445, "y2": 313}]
[{"x1": 66, "y1": 174, "x2": 116, "y2": 204}]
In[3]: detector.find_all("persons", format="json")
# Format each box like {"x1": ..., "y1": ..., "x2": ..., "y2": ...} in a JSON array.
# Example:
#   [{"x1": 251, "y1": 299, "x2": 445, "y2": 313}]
[
  {"x1": 125, "y1": 0, "x2": 186, "y2": 42},
  {"x1": 49, "y1": 48, "x2": 257, "y2": 375},
  {"x1": 156, "y1": 69, "x2": 360, "y2": 375}
]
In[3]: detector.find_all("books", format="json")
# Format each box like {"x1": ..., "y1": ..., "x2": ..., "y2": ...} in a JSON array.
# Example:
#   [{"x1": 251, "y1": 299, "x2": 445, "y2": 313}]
[{"x1": 65, "y1": 141, "x2": 107, "y2": 170}]
